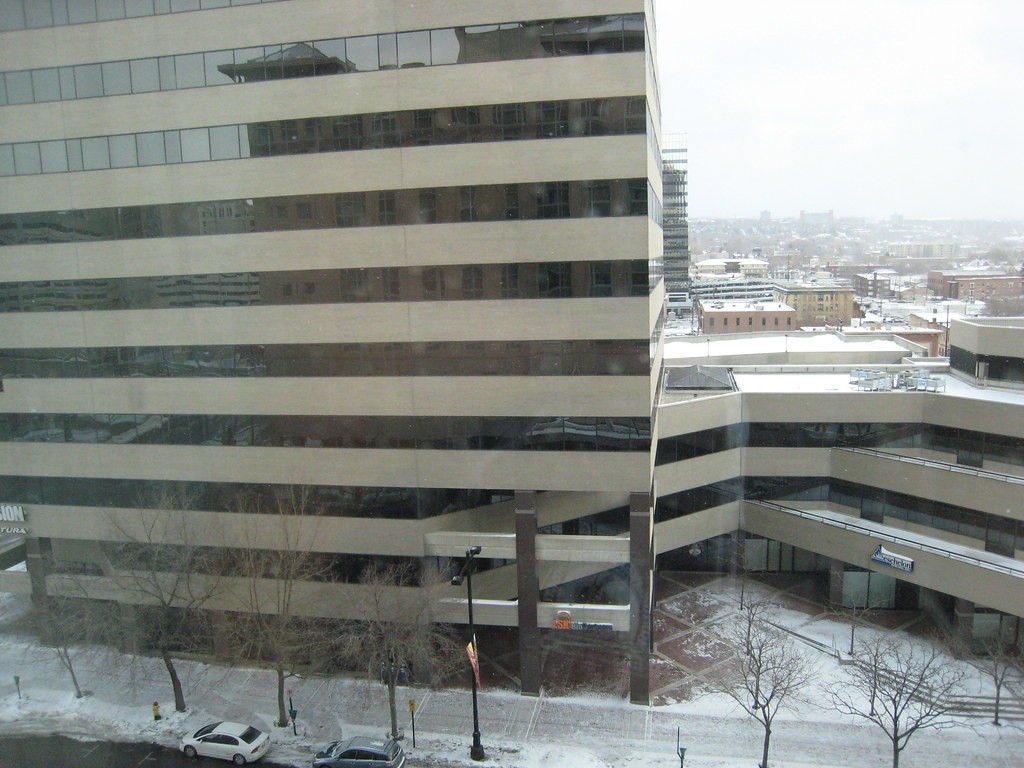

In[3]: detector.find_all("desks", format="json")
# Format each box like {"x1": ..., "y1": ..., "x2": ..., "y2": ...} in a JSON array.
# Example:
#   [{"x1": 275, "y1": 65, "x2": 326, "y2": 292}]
[
  {"x1": 857, "y1": 378, "x2": 892, "y2": 391},
  {"x1": 850, "y1": 370, "x2": 887, "y2": 383},
  {"x1": 899, "y1": 369, "x2": 930, "y2": 382},
  {"x1": 906, "y1": 377, "x2": 947, "y2": 393}
]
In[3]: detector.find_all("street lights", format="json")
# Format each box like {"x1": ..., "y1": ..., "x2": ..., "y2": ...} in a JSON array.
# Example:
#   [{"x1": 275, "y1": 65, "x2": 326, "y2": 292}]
[{"x1": 449, "y1": 544, "x2": 485, "y2": 761}]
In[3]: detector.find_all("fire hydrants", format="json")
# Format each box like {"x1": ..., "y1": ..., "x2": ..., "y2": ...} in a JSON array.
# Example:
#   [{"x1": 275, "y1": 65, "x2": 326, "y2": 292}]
[{"x1": 152, "y1": 701, "x2": 161, "y2": 721}]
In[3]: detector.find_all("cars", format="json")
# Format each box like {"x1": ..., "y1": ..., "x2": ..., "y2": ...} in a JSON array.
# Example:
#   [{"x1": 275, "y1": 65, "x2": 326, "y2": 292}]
[
  {"x1": 313, "y1": 735, "x2": 406, "y2": 768},
  {"x1": 180, "y1": 720, "x2": 271, "y2": 766}
]
